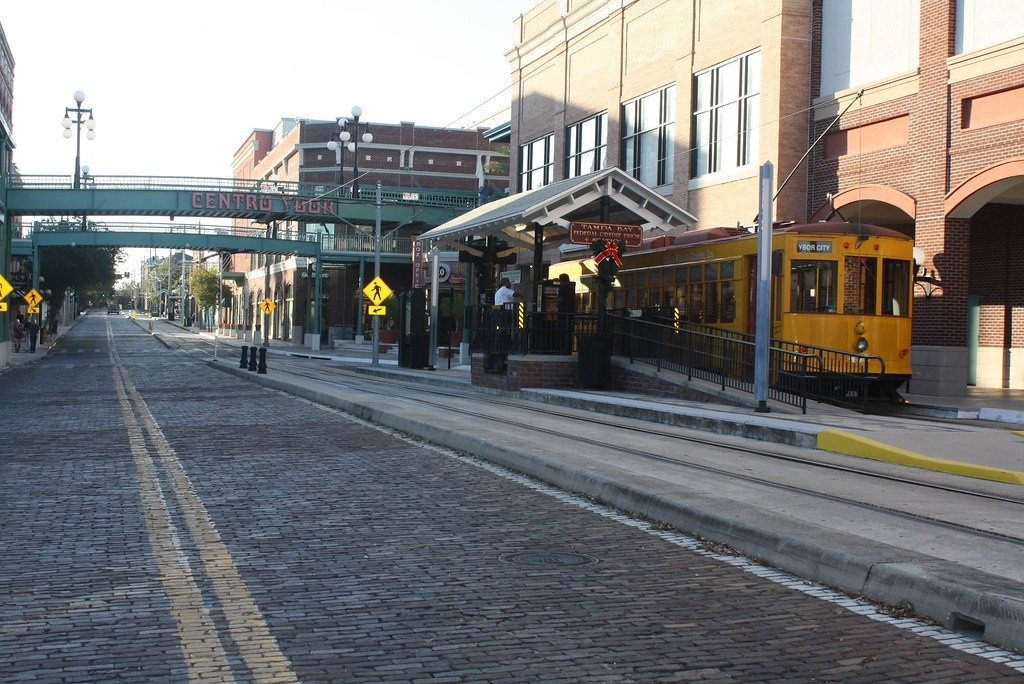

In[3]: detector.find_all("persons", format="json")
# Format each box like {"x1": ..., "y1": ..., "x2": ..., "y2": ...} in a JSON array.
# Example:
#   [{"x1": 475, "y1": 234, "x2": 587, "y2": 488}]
[
  {"x1": 17, "y1": 311, "x2": 24, "y2": 325},
  {"x1": 13, "y1": 319, "x2": 23, "y2": 353},
  {"x1": 555, "y1": 275, "x2": 576, "y2": 321},
  {"x1": 29, "y1": 319, "x2": 39, "y2": 353},
  {"x1": 26, "y1": 312, "x2": 31, "y2": 328},
  {"x1": 495, "y1": 278, "x2": 524, "y2": 311}
]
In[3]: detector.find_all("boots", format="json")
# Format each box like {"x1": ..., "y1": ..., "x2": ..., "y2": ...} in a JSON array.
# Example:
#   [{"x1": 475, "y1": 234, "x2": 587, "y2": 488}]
[
  {"x1": 15, "y1": 344, "x2": 18, "y2": 353},
  {"x1": 18, "y1": 345, "x2": 20, "y2": 352}
]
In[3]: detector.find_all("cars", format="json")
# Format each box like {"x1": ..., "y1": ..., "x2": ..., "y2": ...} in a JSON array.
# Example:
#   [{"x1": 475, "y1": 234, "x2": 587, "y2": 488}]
[{"x1": 108, "y1": 304, "x2": 120, "y2": 315}]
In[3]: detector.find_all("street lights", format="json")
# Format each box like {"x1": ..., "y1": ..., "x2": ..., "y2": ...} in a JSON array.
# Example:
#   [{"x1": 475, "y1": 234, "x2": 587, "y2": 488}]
[
  {"x1": 339, "y1": 105, "x2": 377, "y2": 199},
  {"x1": 327, "y1": 115, "x2": 356, "y2": 197},
  {"x1": 63, "y1": 91, "x2": 96, "y2": 189},
  {"x1": 81, "y1": 166, "x2": 90, "y2": 231}
]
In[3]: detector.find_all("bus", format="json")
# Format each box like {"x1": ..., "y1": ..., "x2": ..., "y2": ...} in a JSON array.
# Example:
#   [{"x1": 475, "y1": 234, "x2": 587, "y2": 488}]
[{"x1": 544, "y1": 221, "x2": 914, "y2": 404}]
